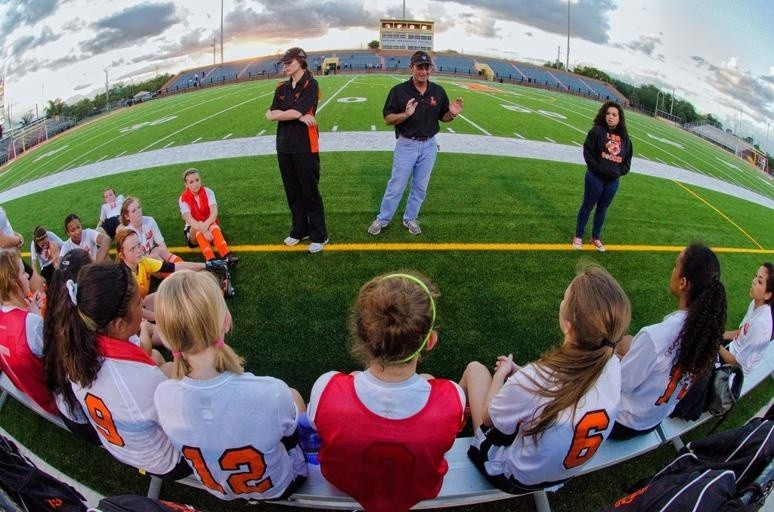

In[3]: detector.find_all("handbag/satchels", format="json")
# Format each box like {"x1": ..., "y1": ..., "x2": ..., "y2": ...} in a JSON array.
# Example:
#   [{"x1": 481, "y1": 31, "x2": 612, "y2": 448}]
[
  {"x1": 669, "y1": 353, "x2": 743, "y2": 421},
  {"x1": 604, "y1": 418, "x2": 774, "y2": 512}
]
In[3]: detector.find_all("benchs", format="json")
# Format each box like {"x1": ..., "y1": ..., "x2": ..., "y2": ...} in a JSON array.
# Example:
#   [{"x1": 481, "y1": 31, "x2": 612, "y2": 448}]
[{"x1": 0, "y1": 341, "x2": 774, "y2": 512}]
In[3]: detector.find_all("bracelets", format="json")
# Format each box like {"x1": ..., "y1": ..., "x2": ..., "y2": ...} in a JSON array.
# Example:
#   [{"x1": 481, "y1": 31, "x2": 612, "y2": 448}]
[{"x1": 447, "y1": 112, "x2": 455, "y2": 119}]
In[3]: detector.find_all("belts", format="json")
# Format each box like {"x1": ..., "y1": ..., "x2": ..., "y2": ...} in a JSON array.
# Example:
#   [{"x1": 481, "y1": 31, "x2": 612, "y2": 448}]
[{"x1": 403, "y1": 135, "x2": 433, "y2": 141}]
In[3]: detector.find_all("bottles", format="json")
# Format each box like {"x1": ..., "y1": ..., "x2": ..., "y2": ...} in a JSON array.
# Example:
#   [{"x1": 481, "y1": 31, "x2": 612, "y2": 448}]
[{"x1": 295, "y1": 411, "x2": 324, "y2": 467}]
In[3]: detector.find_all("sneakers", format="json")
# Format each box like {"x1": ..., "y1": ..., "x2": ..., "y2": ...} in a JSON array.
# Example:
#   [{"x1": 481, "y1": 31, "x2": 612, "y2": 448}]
[
  {"x1": 284, "y1": 233, "x2": 309, "y2": 245},
  {"x1": 573, "y1": 237, "x2": 582, "y2": 247},
  {"x1": 368, "y1": 220, "x2": 388, "y2": 235},
  {"x1": 404, "y1": 219, "x2": 421, "y2": 235},
  {"x1": 590, "y1": 238, "x2": 605, "y2": 251},
  {"x1": 308, "y1": 238, "x2": 329, "y2": 253}
]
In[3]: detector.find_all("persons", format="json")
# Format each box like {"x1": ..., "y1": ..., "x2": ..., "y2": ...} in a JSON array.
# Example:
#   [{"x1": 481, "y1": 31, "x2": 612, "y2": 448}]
[
  {"x1": 361, "y1": 51, "x2": 463, "y2": 234},
  {"x1": 151, "y1": 269, "x2": 306, "y2": 502},
  {"x1": 307, "y1": 267, "x2": 465, "y2": 509},
  {"x1": 715, "y1": 259, "x2": 772, "y2": 375},
  {"x1": 459, "y1": 258, "x2": 631, "y2": 491},
  {"x1": 0, "y1": 168, "x2": 240, "y2": 483},
  {"x1": 265, "y1": 49, "x2": 330, "y2": 253},
  {"x1": 573, "y1": 100, "x2": 634, "y2": 252},
  {"x1": 608, "y1": 240, "x2": 726, "y2": 443}
]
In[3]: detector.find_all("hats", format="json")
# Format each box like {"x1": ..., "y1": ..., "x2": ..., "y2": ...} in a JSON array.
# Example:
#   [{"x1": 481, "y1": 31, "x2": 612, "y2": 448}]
[
  {"x1": 277, "y1": 48, "x2": 306, "y2": 65},
  {"x1": 411, "y1": 50, "x2": 433, "y2": 65}
]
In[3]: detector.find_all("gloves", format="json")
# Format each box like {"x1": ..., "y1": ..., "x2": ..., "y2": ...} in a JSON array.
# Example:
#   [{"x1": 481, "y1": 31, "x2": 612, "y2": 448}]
[{"x1": 205, "y1": 259, "x2": 228, "y2": 273}]
